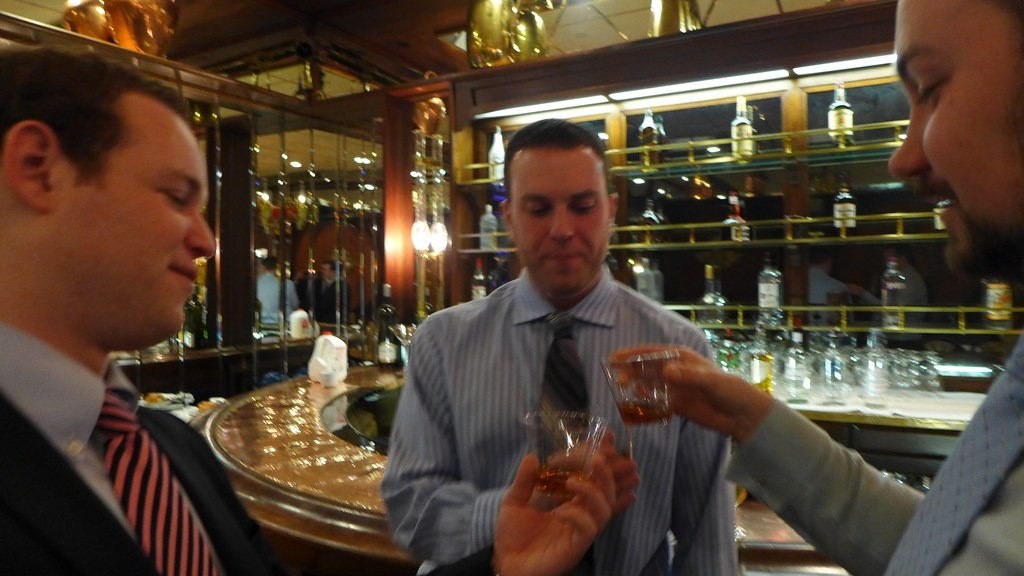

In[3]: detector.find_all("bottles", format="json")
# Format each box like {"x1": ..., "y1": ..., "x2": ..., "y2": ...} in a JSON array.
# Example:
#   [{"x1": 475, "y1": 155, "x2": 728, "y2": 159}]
[{"x1": 2, "y1": 1, "x2": 1024, "y2": 491}]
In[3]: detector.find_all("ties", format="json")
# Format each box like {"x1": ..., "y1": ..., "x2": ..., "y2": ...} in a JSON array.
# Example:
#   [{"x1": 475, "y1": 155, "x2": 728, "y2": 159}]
[
  {"x1": 88, "y1": 393, "x2": 225, "y2": 576},
  {"x1": 536, "y1": 313, "x2": 595, "y2": 576},
  {"x1": 884, "y1": 329, "x2": 1024, "y2": 576}
]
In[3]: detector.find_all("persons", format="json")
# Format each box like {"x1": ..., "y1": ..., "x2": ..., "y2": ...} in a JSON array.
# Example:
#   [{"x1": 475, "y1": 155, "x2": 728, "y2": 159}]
[
  {"x1": 1, "y1": 52, "x2": 641, "y2": 576},
  {"x1": 380, "y1": 118, "x2": 740, "y2": 576},
  {"x1": 608, "y1": 0, "x2": 1024, "y2": 576},
  {"x1": 258, "y1": 253, "x2": 352, "y2": 324}
]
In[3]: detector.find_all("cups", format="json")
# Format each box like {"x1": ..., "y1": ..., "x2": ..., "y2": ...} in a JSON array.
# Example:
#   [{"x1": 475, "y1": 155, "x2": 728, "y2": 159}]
[
  {"x1": 521, "y1": 399, "x2": 606, "y2": 499},
  {"x1": 597, "y1": 348, "x2": 681, "y2": 429}
]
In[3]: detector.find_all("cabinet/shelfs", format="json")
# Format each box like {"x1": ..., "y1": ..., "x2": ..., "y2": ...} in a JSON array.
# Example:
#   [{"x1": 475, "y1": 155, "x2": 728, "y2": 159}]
[{"x1": 447, "y1": 0, "x2": 1023, "y2": 502}]
